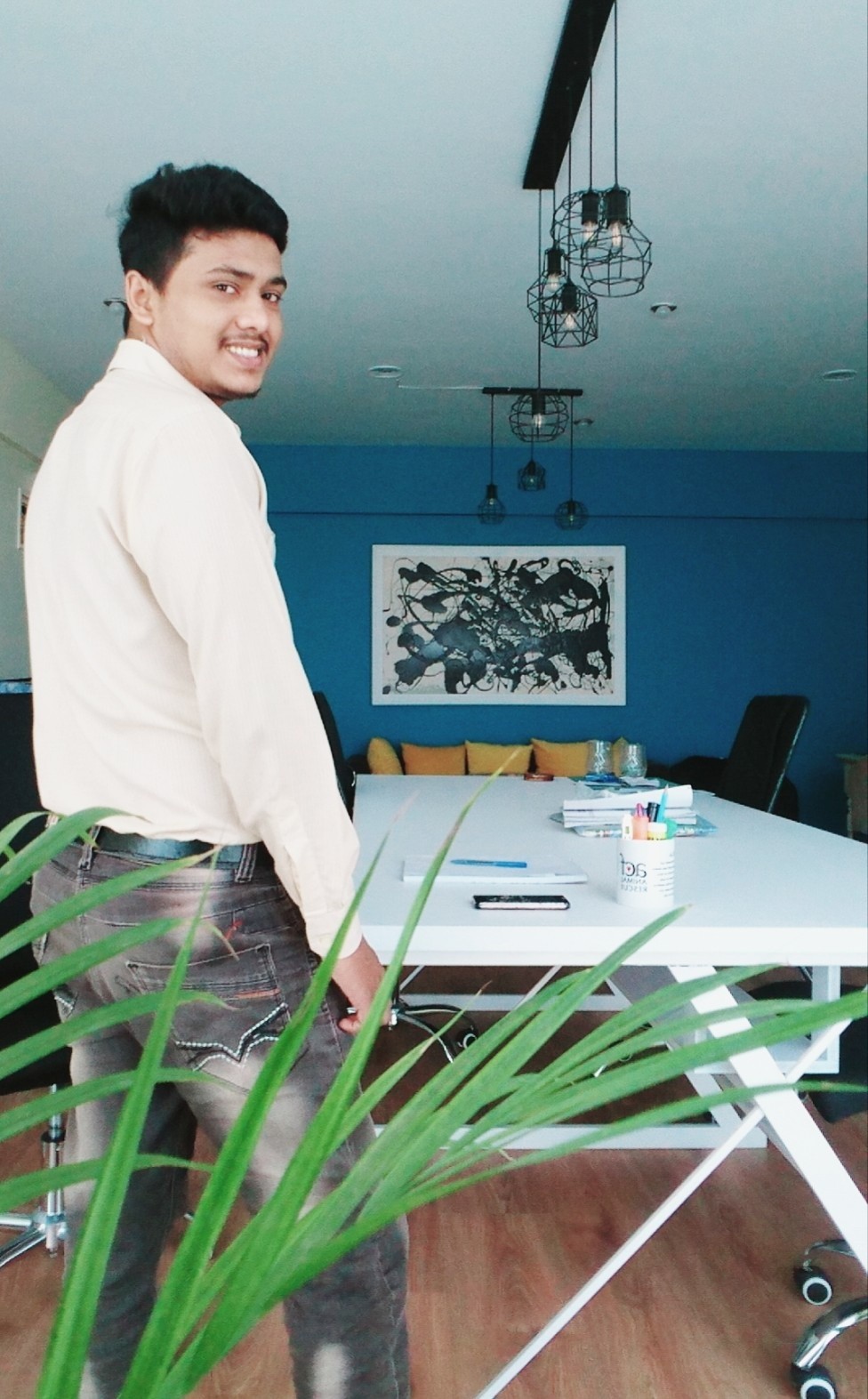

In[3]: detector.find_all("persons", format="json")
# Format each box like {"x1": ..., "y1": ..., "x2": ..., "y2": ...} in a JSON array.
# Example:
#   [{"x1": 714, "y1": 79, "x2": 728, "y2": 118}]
[{"x1": 24, "y1": 161, "x2": 410, "y2": 1399}]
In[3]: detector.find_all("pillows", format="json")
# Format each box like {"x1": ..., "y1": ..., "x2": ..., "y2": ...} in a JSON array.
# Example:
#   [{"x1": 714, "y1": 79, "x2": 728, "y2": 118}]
[
  {"x1": 369, "y1": 737, "x2": 402, "y2": 775},
  {"x1": 464, "y1": 739, "x2": 534, "y2": 778},
  {"x1": 399, "y1": 743, "x2": 466, "y2": 777},
  {"x1": 531, "y1": 736, "x2": 594, "y2": 776}
]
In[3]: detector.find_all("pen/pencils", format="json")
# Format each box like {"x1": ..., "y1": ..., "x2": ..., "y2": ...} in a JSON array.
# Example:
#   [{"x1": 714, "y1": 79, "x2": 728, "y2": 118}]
[
  {"x1": 451, "y1": 859, "x2": 526, "y2": 867},
  {"x1": 636, "y1": 804, "x2": 644, "y2": 816},
  {"x1": 657, "y1": 784, "x2": 668, "y2": 822}
]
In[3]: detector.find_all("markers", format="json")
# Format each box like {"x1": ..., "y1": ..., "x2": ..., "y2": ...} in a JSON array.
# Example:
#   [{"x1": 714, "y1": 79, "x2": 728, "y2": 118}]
[{"x1": 647, "y1": 802, "x2": 660, "y2": 822}]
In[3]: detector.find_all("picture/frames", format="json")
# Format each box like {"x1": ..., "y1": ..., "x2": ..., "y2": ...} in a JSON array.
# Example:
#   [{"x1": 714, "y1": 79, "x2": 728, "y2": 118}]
[
  {"x1": 371, "y1": 543, "x2": 628, "y2": 705},
  {"x1": 17, "y1": 487, "x2": 29, "y2": 548}
]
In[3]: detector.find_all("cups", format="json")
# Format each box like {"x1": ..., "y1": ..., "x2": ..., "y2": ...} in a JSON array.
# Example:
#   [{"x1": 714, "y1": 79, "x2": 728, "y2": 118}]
[
  {"x1": 616, "y1": 837, "x2": 675, "y2": 909},
  {"x1": 586, "y1": 740, "x2": 613, "y2": 776},
  {"x1": 619, "y1": 743, "x2": 647, "y2": 778}
]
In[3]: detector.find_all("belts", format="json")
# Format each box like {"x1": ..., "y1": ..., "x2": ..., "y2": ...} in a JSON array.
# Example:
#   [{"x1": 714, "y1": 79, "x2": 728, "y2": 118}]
[{"x1": 75, "y1": 825, "x2": 271, "y2": 869}]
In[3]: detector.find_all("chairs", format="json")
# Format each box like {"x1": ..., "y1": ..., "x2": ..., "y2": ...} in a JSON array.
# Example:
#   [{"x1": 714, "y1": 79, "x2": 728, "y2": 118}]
[
  {"x1": 709, "y1": 693, "x2": 811, "y2": 812},
  {"x1": 747, "y1": 981, "x2": 868, "y2": 1399},
  {"x1": 0, "y1": 693, "x2": 217, "y2": 1272}
]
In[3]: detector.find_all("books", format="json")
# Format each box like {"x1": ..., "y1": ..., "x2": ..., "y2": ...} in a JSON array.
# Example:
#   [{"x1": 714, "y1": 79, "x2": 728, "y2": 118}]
[
  {"x1": 403, "y1": 850, "x2": 590, "y2": 884},
  {"x1": 561, "y1": 784, "x2": 719, "y2": 838}
]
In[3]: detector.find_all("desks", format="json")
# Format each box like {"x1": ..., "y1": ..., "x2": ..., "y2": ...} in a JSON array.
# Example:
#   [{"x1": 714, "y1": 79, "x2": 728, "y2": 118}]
[{"x1": 350, "y1": 760, "x2": 868, "y2": 1399}]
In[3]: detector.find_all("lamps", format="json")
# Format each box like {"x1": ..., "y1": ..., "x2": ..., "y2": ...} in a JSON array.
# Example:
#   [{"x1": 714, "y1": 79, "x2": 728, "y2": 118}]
[{"x1": 478, "y1": 0, "x2": 652, "y2": 531}]
